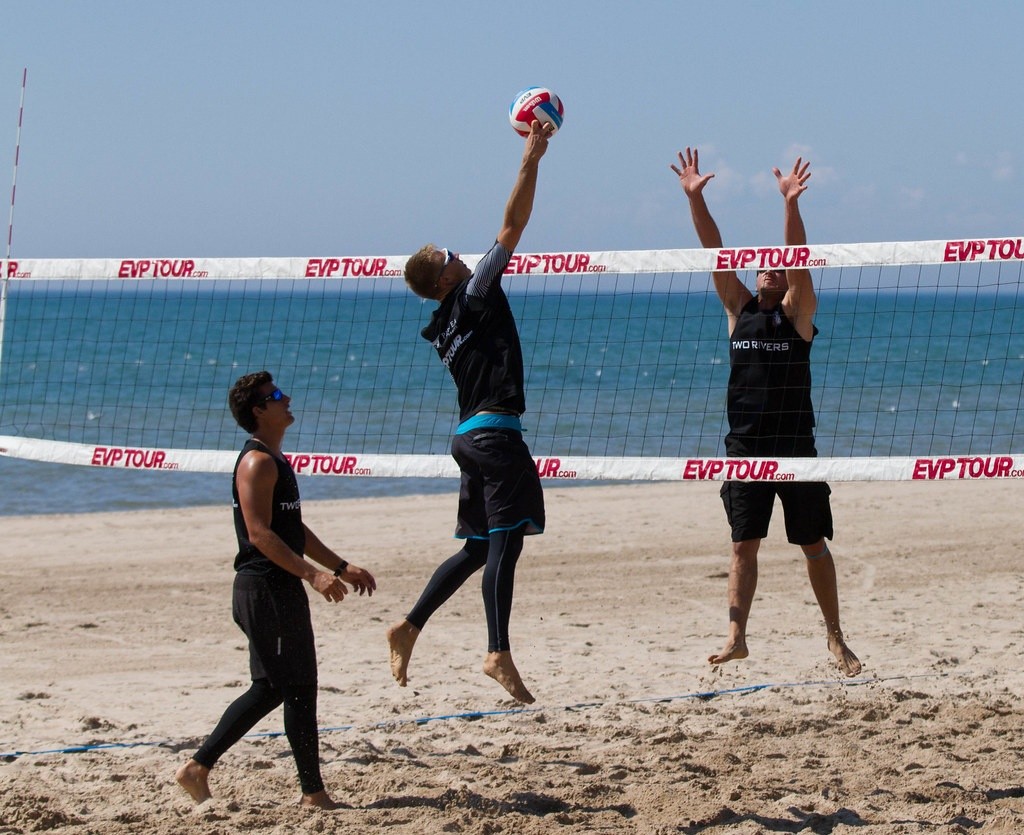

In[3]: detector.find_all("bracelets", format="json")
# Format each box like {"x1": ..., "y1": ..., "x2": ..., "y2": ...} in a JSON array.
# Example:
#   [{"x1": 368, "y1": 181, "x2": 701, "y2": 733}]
[{"x1": 333, "y1": 559, "x2": 348, "y2": 577}]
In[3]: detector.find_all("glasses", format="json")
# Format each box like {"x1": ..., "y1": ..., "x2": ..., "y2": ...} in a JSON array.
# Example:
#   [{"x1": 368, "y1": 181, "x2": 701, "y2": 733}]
[
  {"x1": 264, "y1": 388, "x2": 283, "y2": 401},
  {"x1": 434, "y1": 248, "x2": 455, "y2": 287},
  {"x1": 759, "y1": 270, "x2": 785, "y2": 273}
]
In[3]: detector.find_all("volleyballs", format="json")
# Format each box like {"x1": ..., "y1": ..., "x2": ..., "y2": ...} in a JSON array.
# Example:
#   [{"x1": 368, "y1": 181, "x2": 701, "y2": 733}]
[{"x1": 509, "y1": 86, "x2": 565, "y2": 139}]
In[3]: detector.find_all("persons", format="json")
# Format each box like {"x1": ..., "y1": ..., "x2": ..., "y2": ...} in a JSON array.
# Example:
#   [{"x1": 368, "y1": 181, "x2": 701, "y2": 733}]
[
  {"x1": 385, "y1": 120, "x2": 553, "y2": 706},
  {"x1": 670, "y1": 146, "x2": 862, "y2": 677},
  {"x1": 175, "y1": 372, "x2": 378, "y2": 812}
]
